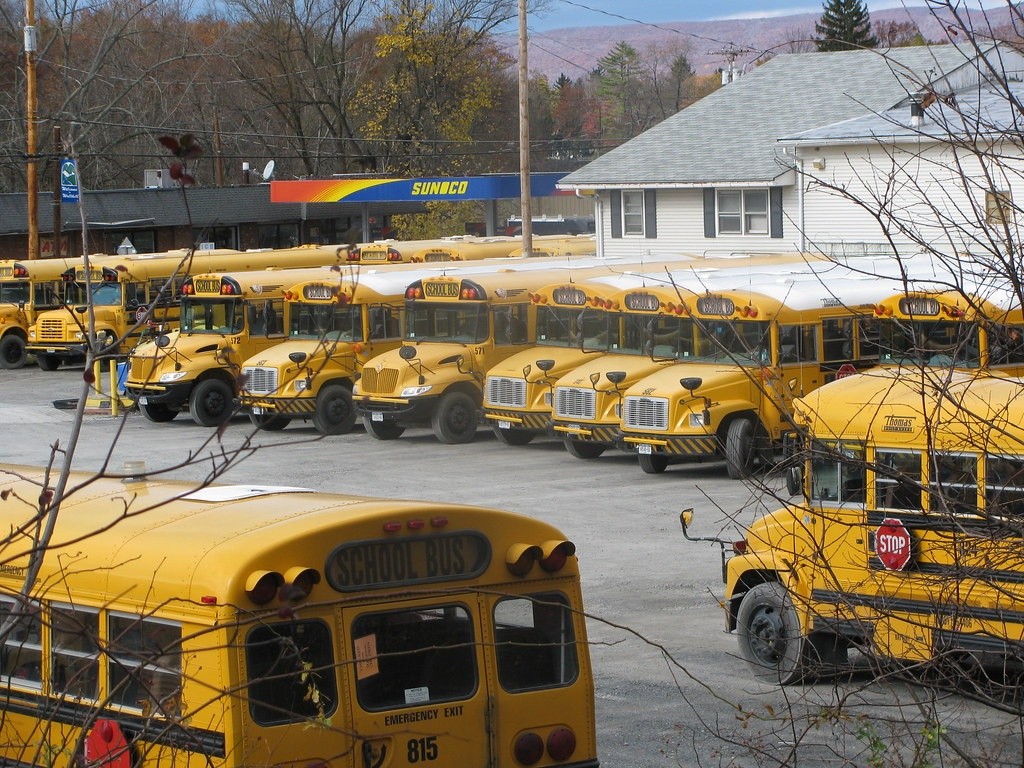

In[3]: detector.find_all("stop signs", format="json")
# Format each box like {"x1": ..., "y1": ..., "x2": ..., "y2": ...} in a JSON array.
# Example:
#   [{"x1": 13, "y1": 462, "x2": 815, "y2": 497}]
[{"x1": 874, "y1": 517, "x2": 911, "y2": 571}]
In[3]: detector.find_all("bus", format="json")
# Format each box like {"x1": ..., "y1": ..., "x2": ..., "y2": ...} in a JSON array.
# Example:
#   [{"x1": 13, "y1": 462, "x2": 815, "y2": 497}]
[
  {"x1": 0, "y1": 232, "x2": 1024, "y2": 480},
  {"x1": 679, "y1": 360, "x2": 1024, "y2": 686},
  {"x1": 0, "y1": 465, "x2": 601, "y2": 767}
]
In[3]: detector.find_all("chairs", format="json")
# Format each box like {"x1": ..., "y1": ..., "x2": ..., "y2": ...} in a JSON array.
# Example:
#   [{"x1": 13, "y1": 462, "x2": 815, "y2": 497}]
[
  {"x1": 837, "y1": 468, "x2": 1023, "y2": 517},
  {"x1": 6, "y1": 607, "x2": 562, "y2": 731}
]
